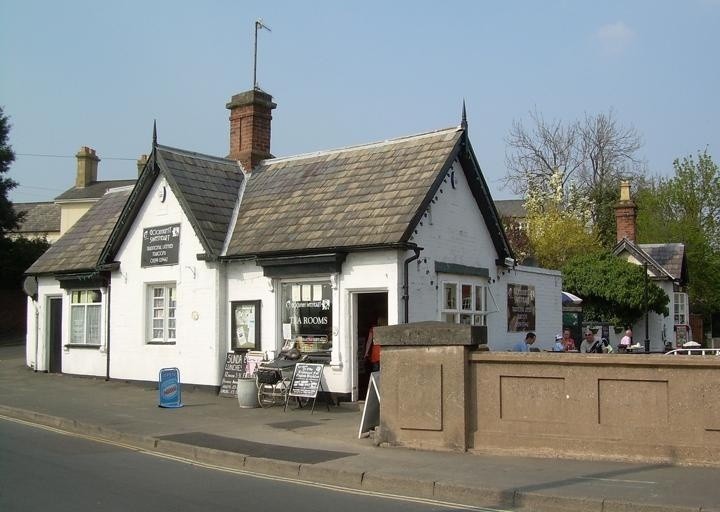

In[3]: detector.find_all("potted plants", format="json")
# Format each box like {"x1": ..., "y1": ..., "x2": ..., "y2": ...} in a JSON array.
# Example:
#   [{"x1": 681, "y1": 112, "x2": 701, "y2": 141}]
[{"x1": 228, "y1": 300, "x2": 261, "y2": 352}]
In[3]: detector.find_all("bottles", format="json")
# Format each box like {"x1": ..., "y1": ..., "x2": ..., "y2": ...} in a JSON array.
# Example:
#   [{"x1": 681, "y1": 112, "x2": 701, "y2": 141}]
[{"x1": 555, "y1": 334, "x2": 564, "y2": 340}]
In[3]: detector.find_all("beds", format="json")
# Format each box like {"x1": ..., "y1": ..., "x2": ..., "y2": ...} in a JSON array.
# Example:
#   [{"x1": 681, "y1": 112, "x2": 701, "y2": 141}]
[{"x1": 561, "y1": 290, "x2": 584, "y2": 305}]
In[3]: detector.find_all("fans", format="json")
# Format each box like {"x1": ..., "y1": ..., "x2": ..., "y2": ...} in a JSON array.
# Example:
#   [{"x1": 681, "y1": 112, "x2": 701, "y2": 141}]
[
  {"x1": 288, "y1": 363, "x2": 324, "y2": 399},
  {"x1": 218, "y1": 353, "x2": 247, "y2": 398}
]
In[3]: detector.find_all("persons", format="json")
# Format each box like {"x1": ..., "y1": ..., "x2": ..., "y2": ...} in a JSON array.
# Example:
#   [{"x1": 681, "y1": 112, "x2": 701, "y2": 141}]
[
  {"x1": 508, "y1": 332, "x2": 537, "y2": 353},
  {"x1": 561, "y1": 327, "x2": 577, "y2": 350},
  {"x1": 362, "y1": 313, "x2": 389, "y2": 372},
  {"x1": 579, "y1": 328, "x2": 606, "y2": 353},
  {"x1": 620, "y1": 328, "x2": 633, "y2": 353},
  {"x1": 553, "y1": 333, "x2": 566, "y2": 352},
  {"x1": 601, "y1": 335, "x2": 616, "y2": 353}
]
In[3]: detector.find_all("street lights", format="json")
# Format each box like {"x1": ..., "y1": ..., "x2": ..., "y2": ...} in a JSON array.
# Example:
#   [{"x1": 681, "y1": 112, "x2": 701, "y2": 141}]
[{"x1": 257, "y1": 369, "x2": 281, "y2": 385}]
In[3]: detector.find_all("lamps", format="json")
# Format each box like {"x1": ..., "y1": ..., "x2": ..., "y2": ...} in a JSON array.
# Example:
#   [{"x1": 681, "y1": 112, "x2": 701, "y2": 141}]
[{"x1": 617, "y1": 344, "x2": 627, "y2": 353}]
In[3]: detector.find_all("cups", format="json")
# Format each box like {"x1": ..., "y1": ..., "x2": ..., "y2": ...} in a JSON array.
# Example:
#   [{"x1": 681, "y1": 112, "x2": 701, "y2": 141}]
[{"x1": 585, "y1": 334, "x2": 591, "y2": 336}]
